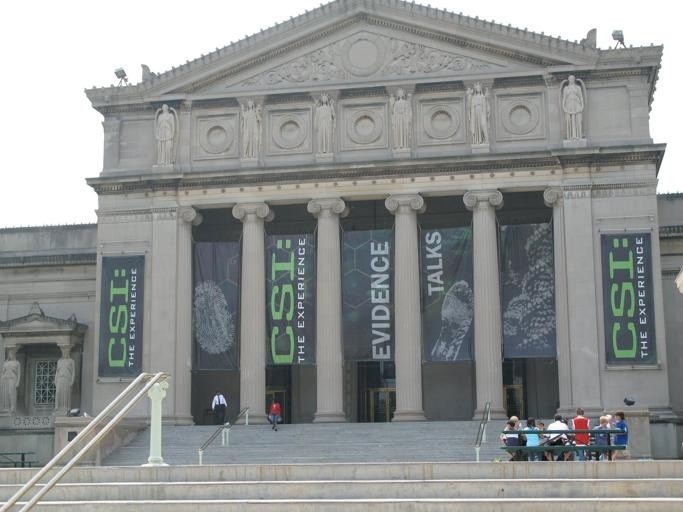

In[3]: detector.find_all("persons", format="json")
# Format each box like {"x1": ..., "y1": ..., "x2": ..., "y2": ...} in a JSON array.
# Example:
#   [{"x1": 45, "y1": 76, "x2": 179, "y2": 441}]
[
  {"x1": 271, "y1": 399, "x2": 280, "y2": 431},
  {"x1": 499, "y1": 407, "x2": 628, "y2": 461},
  {"x1": 211, "y1": 391, "x2": 227, "y2": 425}
]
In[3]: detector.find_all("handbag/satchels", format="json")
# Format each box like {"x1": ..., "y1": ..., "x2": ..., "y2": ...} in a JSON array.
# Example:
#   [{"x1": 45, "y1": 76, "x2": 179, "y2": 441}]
[
  {"x1": 278, "y1": 416, "x2": 282, "y2": 422},
  {"x1": 548, "y1": 435, "x2": 564, "y2": 445}
]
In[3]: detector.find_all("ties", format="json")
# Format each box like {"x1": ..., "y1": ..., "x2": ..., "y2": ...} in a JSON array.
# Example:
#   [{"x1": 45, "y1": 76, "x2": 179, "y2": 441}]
[{"x1": 218, "y1": 395, "x2": 220, "y2": 405}]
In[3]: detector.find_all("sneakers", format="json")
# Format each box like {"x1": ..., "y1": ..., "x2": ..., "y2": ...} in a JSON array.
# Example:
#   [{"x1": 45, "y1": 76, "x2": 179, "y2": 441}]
[{"x1": 272, "y1": 424, "x2": 277, "y2": 431}]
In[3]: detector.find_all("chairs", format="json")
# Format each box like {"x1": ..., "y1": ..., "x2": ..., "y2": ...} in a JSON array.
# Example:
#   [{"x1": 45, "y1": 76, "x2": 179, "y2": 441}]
[
  {"x1": 0, "y1": 451, "x2": 35, "y2": 467},
  {"x1": 499, "y1": 428, "x2": 627, "y2": 461}
]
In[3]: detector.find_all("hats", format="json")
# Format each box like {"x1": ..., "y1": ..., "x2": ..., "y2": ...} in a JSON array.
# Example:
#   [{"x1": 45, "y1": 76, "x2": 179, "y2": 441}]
[{"x1": 510, "y1": 416, "x2": 518, "y2": 421}]
